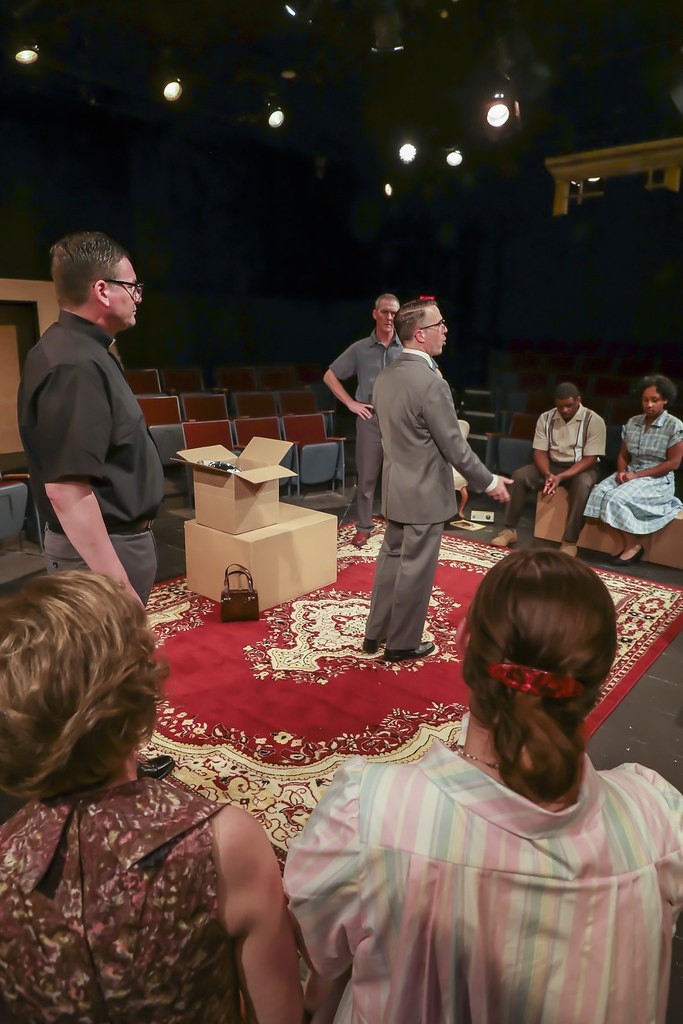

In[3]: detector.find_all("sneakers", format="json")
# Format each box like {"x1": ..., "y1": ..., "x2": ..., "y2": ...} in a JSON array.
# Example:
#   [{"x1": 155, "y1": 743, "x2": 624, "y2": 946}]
[
  {"x1": 558, "y1": 541, "x2": 578, "y2": 557},
  {"x1": 490, "y1": 529, "x2": 517, "y2": 547}
]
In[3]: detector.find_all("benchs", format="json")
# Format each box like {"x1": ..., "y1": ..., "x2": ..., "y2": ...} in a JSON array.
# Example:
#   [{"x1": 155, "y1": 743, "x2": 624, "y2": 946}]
[{"x1": 533, "y1": 486, "x2": 683, "y2": 570}]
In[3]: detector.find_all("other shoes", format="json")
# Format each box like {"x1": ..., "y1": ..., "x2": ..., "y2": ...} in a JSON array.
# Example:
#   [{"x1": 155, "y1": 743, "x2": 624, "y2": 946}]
[{"x1": 614, "y1": 545, "x2": 643, "y2": 566}]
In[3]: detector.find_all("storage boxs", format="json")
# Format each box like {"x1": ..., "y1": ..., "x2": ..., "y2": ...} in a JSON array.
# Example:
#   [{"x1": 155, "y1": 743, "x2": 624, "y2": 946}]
[
  {"x1": 184, "y1": 502, "x2": 338, "y2": 613},
  {"x1": 178, "y1": 432, "x2": 302, "y2": 534}
]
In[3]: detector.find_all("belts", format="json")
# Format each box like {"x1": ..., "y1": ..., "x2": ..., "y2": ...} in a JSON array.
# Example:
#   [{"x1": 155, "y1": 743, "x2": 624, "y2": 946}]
[{"x1": 47, "y1": 519, "x2": 153, "y2": 535}]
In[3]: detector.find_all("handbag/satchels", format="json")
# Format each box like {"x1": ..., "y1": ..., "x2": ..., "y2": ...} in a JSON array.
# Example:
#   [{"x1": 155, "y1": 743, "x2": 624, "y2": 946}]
[{"x1": 220, "y1": 564, "x2": 259, "y2": 622}]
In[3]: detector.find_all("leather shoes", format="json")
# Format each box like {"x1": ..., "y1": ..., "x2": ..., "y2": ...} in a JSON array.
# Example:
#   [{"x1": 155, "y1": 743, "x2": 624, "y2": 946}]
[
  {"x1": 137, "y1": 756, "x2": 175, "y2": 781},
  {"x1": 352, "y1": 531, "x2": 370, "y2": 546},
  {"x1": 383, "y1": 642, "x2": 435, "y2": 661},
  {"x1": 363, "y1": 638, "x2": 386, "y2": 653}
]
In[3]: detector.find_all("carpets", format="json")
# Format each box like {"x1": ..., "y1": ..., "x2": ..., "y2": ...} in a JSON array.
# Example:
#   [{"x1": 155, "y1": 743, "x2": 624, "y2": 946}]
[{"x1": 130, "y1": 516, "x2": 683, "y2": 880}]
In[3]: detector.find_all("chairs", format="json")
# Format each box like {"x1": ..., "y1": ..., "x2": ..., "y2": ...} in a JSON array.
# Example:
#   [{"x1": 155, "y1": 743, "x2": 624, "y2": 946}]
[
  {"x1": 113, "y1": 351, "x2": 348, "y2": 515},
  {"x1": 486, "y1": 350, "x2": 682, "y2": 479},
  {"x1": 0, "y1": 471, "x2": 46, "y2": 555}
]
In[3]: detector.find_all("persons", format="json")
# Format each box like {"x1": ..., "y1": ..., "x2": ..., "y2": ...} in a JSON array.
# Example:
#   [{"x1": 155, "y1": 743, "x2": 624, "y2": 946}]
[
  {"x1": 0, "y1": 570, "x2": 304, "y2": 1023},
  {"x1": 490, "y1": 381, "x2": 608, "y2": 557},
  {"x1": 13, "y1": 230, "x2": 175, "y2": 781},
  {"x1": 359, "y1": 297, "x2": 513, "y2": 664},
  {"x1": 281, "y1": 545, "x2": 683, "y2": 1024},
  {"x1": 322, "y1": 293, "x2": 442, "y2": 545},
  {"x1": 581, "y1": 375, "x2": 683, "y2": 566}
]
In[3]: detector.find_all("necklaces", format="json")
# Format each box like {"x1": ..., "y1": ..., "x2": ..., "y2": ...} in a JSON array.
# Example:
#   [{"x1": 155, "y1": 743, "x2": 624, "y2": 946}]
[{"x1": 456, "y1": 745, "x2": 502, "y2": 771}]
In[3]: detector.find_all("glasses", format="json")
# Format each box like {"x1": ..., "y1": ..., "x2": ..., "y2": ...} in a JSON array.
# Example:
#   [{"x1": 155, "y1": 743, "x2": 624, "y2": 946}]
[
  {"x1": 93, "y1": 279, "x2": 144, "y2": 300},
  {"x1": 414, "y1": 319, "x2": 446, "y2": 337}
]
